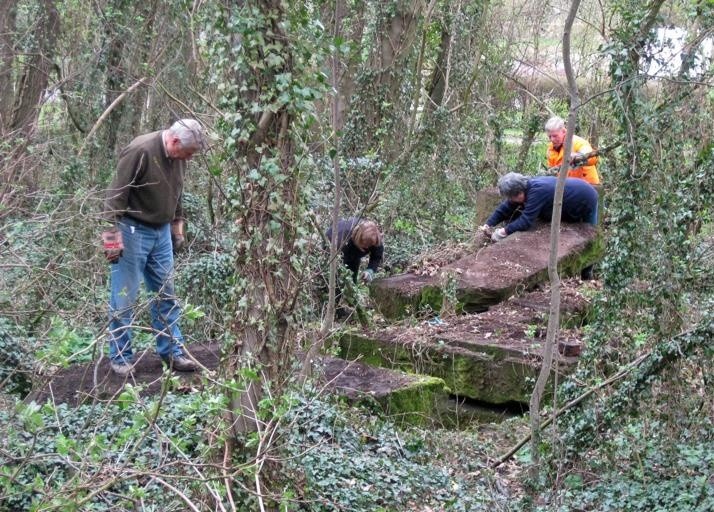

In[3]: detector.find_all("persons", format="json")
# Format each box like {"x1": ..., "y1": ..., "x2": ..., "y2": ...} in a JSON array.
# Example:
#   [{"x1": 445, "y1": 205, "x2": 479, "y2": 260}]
[
  {"x1": 102, "y1": 119, "x2": 205, "y2": 378},
  {"x1": 311, "y1": 217, "x2": 384, "y2": 321},
  {"x1": 545, "y1": 115, "x2": 601, "y2": 191},
  {"x1": 483, "y1": 172, "x2": 599, "y2": 283}
]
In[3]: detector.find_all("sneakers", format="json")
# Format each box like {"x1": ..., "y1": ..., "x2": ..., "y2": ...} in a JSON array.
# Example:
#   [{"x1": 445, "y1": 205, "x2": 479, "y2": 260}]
[
  {"x1": 165, "y1": 349, "x2": 197, "y2": 371},
  {"x1": 111, "y1": 361, "x2": 136, "y2": 378}
]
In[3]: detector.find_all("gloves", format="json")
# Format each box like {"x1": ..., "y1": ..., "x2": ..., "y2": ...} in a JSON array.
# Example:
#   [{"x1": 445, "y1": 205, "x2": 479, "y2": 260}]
[
  {"x1": 171, "y1": 219, "x2": 186, "y2": 254},
  {"x1": 358, "y1": 268, "x2": 374, "y2": 283},
  {"x1": 490, "y1": 228, "x2": 506, "y2": 242},
  {"x1": 101, "y1": 232, "x2": 125, "y2": 265}
]
